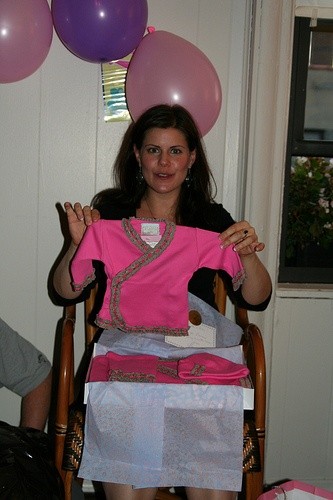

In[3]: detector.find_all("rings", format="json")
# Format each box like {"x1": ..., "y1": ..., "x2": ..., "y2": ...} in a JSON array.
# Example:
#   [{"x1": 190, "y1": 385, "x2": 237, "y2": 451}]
[{"x1": 243, "y1": 229, "x2": 249, "y2": 241}]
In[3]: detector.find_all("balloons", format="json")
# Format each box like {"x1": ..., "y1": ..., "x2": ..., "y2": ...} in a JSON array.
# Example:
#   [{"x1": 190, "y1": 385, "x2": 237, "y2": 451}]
[
  {"x1": 123, "y1": 26, "x2": 222, "y2": 138},
  {"x1": 0, "y1": 0, "x2": 53, "y2": 84},
  {"x1": 52, "y1": 1, "x2": 149, "y2": 66}
]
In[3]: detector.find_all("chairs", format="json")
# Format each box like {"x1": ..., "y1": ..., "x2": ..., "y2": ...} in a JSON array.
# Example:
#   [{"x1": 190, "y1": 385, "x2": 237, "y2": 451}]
[{"x1": 56, "y1": 268, "x2": 266, "y2": 500}]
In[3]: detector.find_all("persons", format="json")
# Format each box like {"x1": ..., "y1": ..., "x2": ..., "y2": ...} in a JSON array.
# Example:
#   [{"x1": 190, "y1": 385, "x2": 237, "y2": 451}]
[
  {"x1": 0, "y1": 319, "x2": 54, "y2": 432},
  {"x1": 52, "y1": 102, "x2": 272, "y2": 500}
]
